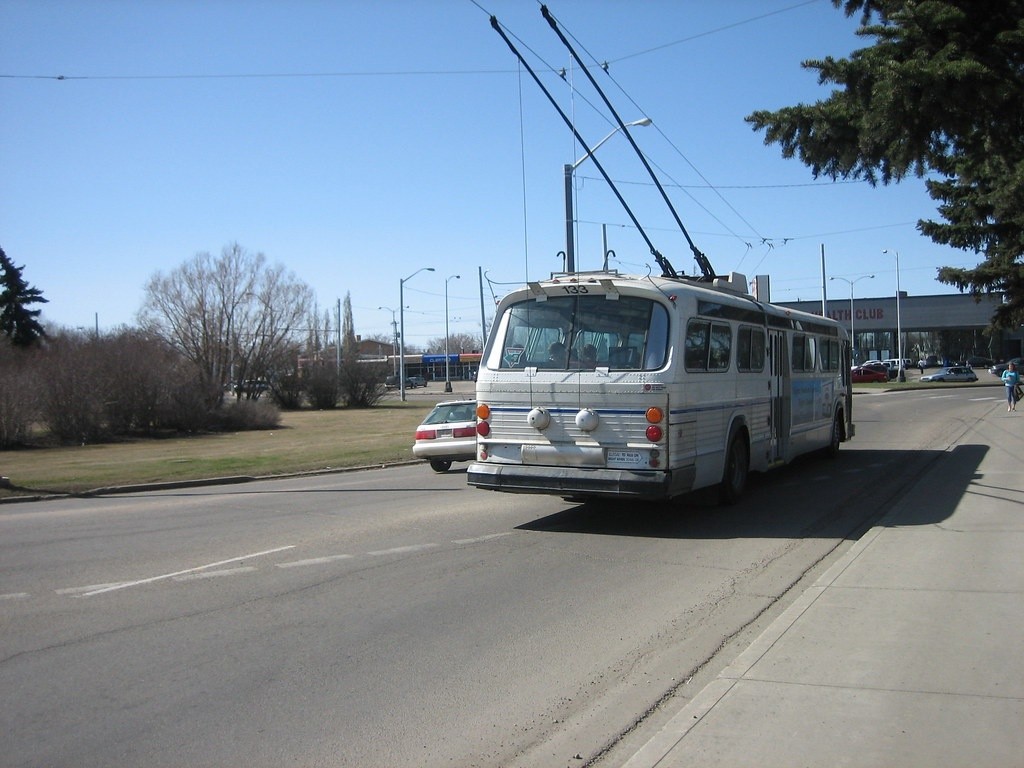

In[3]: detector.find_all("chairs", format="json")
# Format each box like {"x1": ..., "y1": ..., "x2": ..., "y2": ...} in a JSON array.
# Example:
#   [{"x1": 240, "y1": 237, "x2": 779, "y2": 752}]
[
  {"x1": 449, "y1": 412, "x2": 466, "y2": 420},
  {"x1": 510, "y1": 342, "x2": 747, "y2": 370}
]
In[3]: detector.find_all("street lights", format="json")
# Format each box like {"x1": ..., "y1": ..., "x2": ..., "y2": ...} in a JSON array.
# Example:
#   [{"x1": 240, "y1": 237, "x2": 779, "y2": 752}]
[
  {"x1": 378, "y1": 306, "x2": 409, "y2": 375},
  {"x1": 565, "y1": 118, "x2": 651, "y2": 274},
  {"x1": 829, "y1": 275, "x2": 875, "y2": 367},
  {"x1": 400, "y1": 267, "x2": 435, "y2": 402},
  {"x1": 445, "y1": 275, "x2": 461, "y2": 392},
  {"x1": 246, "y1": 292, "x2": 273, "y2": 345},
  {"x1": 882, "y1": 249, "x2": 906, "y2": 383}
]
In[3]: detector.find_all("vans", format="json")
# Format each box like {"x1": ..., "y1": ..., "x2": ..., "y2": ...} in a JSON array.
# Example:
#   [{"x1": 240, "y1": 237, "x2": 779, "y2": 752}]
[{"x1": 861, "y1": 358, "x2": 913, "y2": 378}]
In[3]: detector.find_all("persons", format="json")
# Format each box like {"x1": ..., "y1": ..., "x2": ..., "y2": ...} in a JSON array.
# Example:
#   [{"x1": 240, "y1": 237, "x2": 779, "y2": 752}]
[
  {"x1": 547, "y1": 342, "x2": 566, "y2": 363},
  {"x1": 578, "y1": 344, "x2": 601, "y2": 369},
  {"x1": 510, "y1": 345, "x2": 526, "y2": 368},
  {"x1": 1001, "y1": 362, "x2": 1020, "y2": 411},
  {"x1": 966, "y1": 361, "x2": 972, "y2": 370},
  {"x1": 918, "y1": 358, "x2": 924, "y2": 374}
]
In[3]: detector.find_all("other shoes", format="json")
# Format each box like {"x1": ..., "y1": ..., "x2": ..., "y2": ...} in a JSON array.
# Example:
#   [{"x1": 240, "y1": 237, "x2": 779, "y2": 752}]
[{"x1": 1008, "y1": 408, "x2": 1015, "y2": 412}]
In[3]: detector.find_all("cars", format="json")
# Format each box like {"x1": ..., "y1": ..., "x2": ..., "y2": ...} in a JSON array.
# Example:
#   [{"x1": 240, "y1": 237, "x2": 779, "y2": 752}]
[
  {"x1": 413, "y1": 400, "x2": 478, "y2": 472},
  {"x1": 410, "y1": 377, "x2": 428, "y2": 388},
  {"x1": 955, "y1": 357, "x2": 995, "y2": 369},
  {"x1": 988, "y1": 358, "x2": 1024, "y2": 377},
  {"x1": 851, "y1": 367, "x2": 889, "y2": 383},
  {"x1": 224, "y1": 380, "x2": 269, "y2": 394},
  {"x1": 384, "y1": 376, "x2": 414, "y2": 390},
  {"x1": 919, "y1": 366, "x2": 978, "y2": 382}
]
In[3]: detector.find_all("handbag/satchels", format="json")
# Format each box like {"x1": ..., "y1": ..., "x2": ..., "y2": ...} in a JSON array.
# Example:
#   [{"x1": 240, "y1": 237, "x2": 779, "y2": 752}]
[
  {"x1": 918, "y1": 364, "x2": 920, "y2": 367},
  {"x1": 1012, "y1": 383, "x2": 1024, "y2": 401}
]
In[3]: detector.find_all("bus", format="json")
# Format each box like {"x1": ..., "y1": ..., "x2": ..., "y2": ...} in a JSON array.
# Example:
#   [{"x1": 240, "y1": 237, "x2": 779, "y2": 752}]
[{"x1": 464, "y1": 3, "x2": 857, "y2": 523}]
